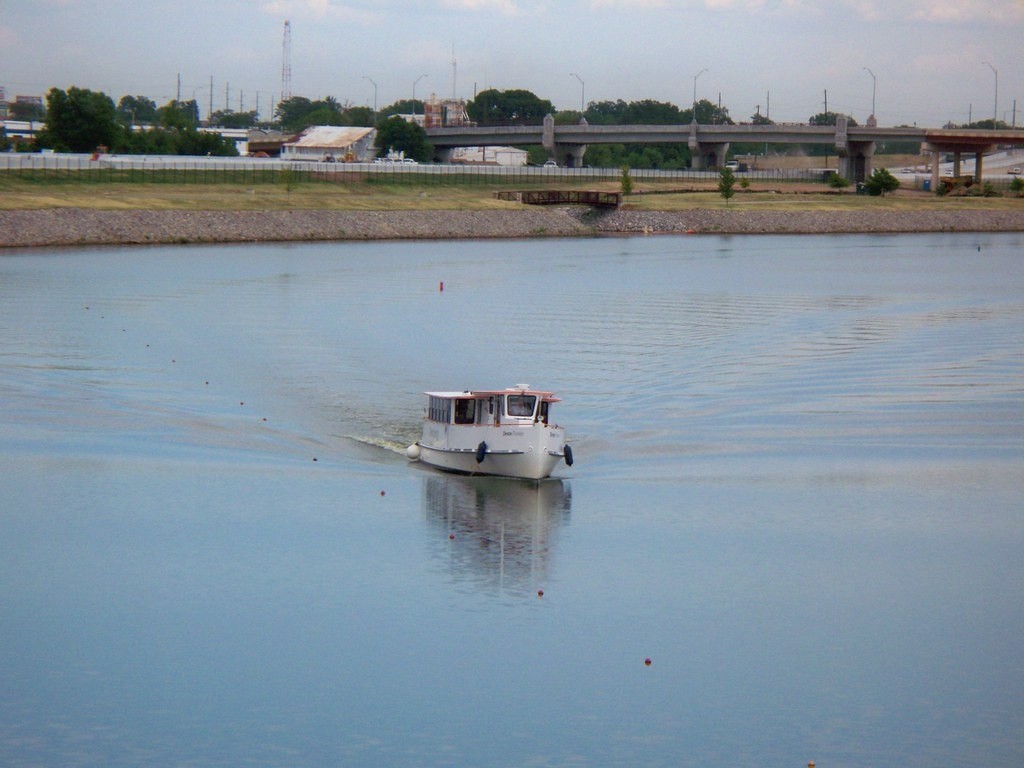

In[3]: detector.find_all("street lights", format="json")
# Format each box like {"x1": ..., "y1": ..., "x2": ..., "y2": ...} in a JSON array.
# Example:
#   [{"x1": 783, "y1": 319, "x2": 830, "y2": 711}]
[
  {"x1": 864, "y1": 67, "x2": 875, "y2": 113},
  {"x1": 413, "y1": 73, "x2": 428, "y2": 116},
  {"x1": 981, "y1": 61, "x2": 998, "y2": 128},
  {"x1": 363, "y1": 76, "x2": 377, "y2": 111},
  {"x1": 569, "y1": 73, "x2": 584, "y2": 119},
  {"x1": 693, "y1": 68, "x2": 707, "y2": 119}
]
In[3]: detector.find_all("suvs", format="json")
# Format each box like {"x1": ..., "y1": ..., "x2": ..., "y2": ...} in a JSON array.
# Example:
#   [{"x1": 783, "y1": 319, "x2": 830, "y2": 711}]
[{"x1": 544, "y1": 160, "x2": 558, "y2": 168}]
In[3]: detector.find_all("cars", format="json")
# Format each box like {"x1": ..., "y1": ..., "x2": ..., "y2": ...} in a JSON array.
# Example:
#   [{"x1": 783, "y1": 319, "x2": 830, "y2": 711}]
[
  {"x1": 902, "y1": 168, "x2": 912, "y2": 173},
  {"x1": 375, "y1": 157, "x2": 415, "y2": 164},
  {"x1": 726, "y1": 160, "x2": 747, "y2": 172},
  {"x1": 946, "y1": 169, "x2": 954, "y2": 174}
]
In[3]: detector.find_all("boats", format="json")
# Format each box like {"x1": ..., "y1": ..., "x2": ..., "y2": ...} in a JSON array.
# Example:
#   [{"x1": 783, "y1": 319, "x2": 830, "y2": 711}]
[{"x1": 413, "y1": 383, "x2": 566, "y2": 478}]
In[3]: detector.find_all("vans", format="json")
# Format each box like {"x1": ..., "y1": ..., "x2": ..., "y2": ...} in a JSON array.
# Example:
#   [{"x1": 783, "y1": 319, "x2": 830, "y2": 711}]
[{"x1": 1008, "y1": 167, "x2": 1020, "y2": 174}]
